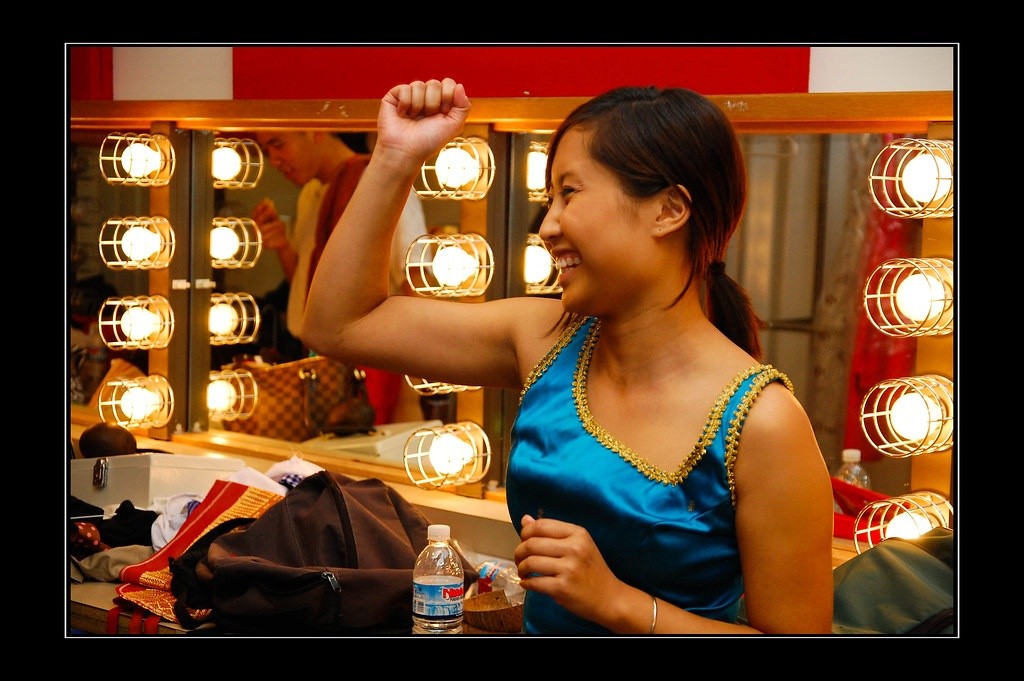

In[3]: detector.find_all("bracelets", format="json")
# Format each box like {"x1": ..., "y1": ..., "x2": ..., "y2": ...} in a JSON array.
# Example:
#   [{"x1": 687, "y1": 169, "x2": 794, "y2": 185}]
[{"x1": 651, "y1": 596, "x2": 658, "y2": 634}]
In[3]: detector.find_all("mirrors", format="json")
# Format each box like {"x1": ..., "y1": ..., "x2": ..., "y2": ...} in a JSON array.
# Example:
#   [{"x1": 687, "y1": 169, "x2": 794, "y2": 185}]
[{"x1": 72, "y1": 90, "x2": 953, "y2": 547}]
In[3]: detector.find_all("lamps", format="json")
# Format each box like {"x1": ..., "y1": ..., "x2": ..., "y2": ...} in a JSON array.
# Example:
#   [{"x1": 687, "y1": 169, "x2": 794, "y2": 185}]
[
  {"x1": 98, "y1": 295, "x2": 175, "y2": 351},
  {"x1": 210, "y1": 136, "x2": 264, "y2": 190},
  {"x1": 523, "y1": 231, "x2": 566, "y2": 295},
  {"x1": 402, "y1": 136, "x2": 495, "y2": 489},
  {"x1": 852, "y1": 137, "x2": 955, "y2": 554},
  {"x1": 208, "y1": 291, "x2": 261, "y2": 346},
  {"x1": 210, "y1": 217, "x2": 262, "y2": 269},
  {"x1": 526, "y1": 140, "x2": 551, "y2": 203},
  {"x1": 206, "y1": 369, "x2": 260, "y2": 424},
  {"x1": 99, "y1": 216, "x2": 174, "y2": 270},
  {"x1": 98, "y1": 374, "x2": 175, "y2": 429},
  {"x1": 99, "y1": 132, "x2": 176, "y2": 188}
]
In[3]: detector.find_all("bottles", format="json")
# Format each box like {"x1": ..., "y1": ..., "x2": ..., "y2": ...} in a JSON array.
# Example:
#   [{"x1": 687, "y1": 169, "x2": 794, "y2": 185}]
[
  {"x1": 411, "y1": 524, "x2": 465, "y2": 634},
  {"x1": 474, "y1": 560, "x2": 526, "y2": 607},
  {"x1": 835, "y1": 449, "x2": 871, "y2": 513}
]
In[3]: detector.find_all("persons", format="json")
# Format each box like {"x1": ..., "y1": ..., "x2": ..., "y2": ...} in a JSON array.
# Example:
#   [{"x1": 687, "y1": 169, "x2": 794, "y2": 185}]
[
  {"x1": 302, "y1": 77, "x2": 834, "y2": 635},
  {"x1": 252, "y1": 127, "x2": 432, "y2": 426}
]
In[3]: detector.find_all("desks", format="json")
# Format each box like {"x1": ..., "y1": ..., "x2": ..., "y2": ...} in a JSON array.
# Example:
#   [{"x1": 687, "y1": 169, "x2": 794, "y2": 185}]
[{"x1": 69, "y1": 582, "x2": 214, "y2": 635}]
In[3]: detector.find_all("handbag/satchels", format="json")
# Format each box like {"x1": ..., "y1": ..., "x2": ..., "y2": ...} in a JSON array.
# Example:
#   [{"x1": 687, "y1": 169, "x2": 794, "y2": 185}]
[
  {"x1": 170, "y1": 469, "x2": 481, "y2": 637},
  {"x1": 221, "y1": 349, "x2": 374, "y2": 442}
]
